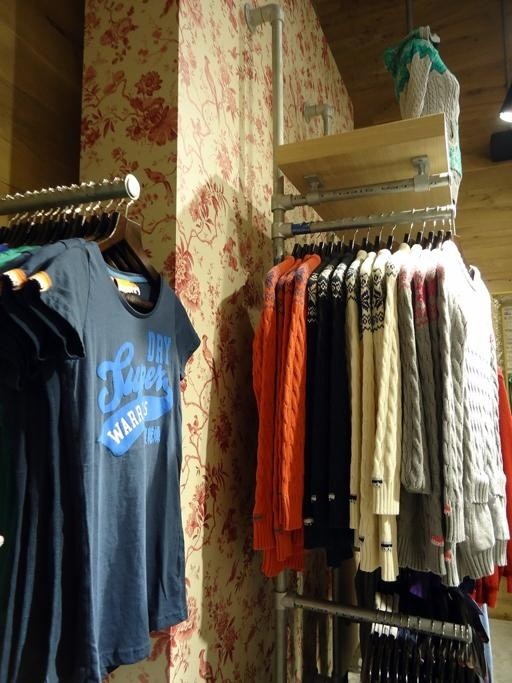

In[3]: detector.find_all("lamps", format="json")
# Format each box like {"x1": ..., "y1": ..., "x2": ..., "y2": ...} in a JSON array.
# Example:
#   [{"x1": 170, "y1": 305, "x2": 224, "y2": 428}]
[{"x1": 498, "y1": 84, "x2": 512, "y2": 123}]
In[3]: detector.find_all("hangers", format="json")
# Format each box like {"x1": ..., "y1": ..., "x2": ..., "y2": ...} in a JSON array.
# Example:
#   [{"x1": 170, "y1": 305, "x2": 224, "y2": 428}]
[
  {"x1": 263, "y1": 203, "x2": 475, "y2": 293},
  {"x1": 0, "y1": 174, "x2": 166, "y2": 311},
  {"x1": 358, "y1": 606, "x2": 481, "y2": 683}
]
